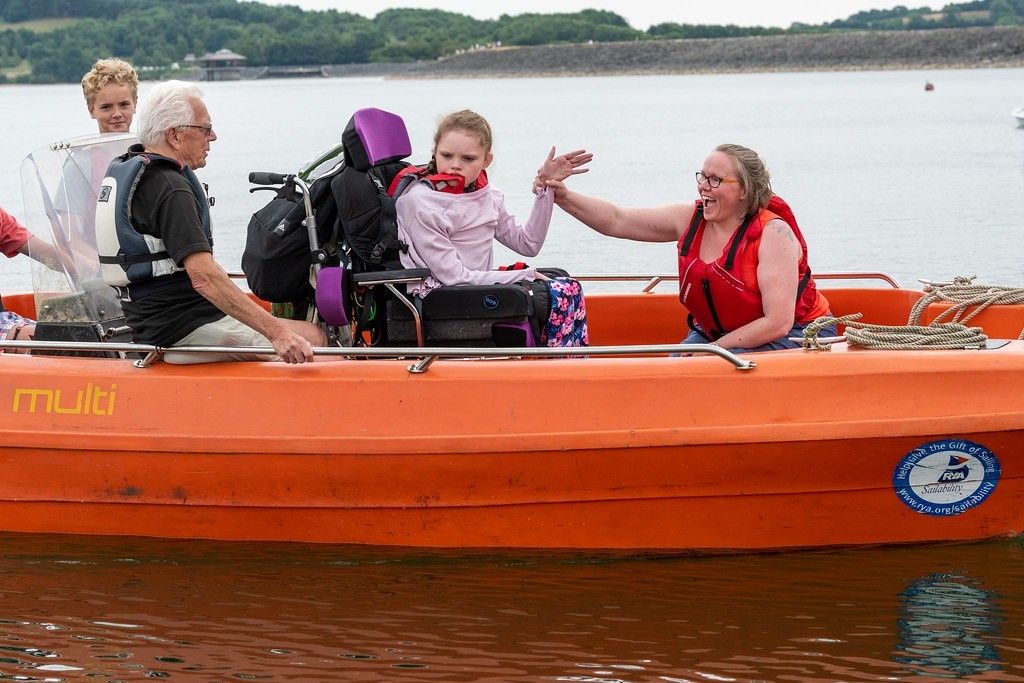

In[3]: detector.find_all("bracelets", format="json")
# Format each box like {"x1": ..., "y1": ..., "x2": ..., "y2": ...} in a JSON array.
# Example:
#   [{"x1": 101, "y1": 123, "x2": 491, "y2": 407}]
[{"x1": 709, "y1": 342, "x2": 718, "y2": 347}]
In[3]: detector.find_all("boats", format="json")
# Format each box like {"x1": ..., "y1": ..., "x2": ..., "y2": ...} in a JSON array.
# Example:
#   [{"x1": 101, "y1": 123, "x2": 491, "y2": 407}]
[{"x1": 0, "y1": 133, "x2": 1024, "y2": 556}]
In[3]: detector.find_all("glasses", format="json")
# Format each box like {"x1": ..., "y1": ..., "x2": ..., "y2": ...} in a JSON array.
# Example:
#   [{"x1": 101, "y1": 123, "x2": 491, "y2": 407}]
[
  {"x1": 695, "y1": 172, "x2": 740, "y2": 188},
  {"x1": 177, "y1": 123, "x2": 212, "y2": 136}
]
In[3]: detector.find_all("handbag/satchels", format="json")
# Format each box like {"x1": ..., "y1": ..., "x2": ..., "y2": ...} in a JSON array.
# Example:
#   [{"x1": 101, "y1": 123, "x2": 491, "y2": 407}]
[{"x1": 242, "y1": 181, "x2": 310, "y2": 303}]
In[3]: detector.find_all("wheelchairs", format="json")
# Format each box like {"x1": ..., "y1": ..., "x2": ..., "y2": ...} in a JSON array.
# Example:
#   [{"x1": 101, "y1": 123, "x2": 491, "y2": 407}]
[{"x1": 249, "y1": 107, "x2": 570, "y2": 359}]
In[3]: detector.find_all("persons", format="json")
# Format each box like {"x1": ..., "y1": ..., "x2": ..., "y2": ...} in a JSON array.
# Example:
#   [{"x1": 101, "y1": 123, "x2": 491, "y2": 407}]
[
  {"x1": 52, "y1": 58, "x2": 139, "y2": 290},
  {"x1": 529, "y1": 143, "x2": 835, "y2": 358},
  {"x1": 94, "y1": 80, "x2": 345, "y2": 365},
  {"x1": 0, "y1": 209, "x2": 85, "y2": 355},
  {"x1": 395, "y1": 109, "x2": 592, "y2": 360}
]
